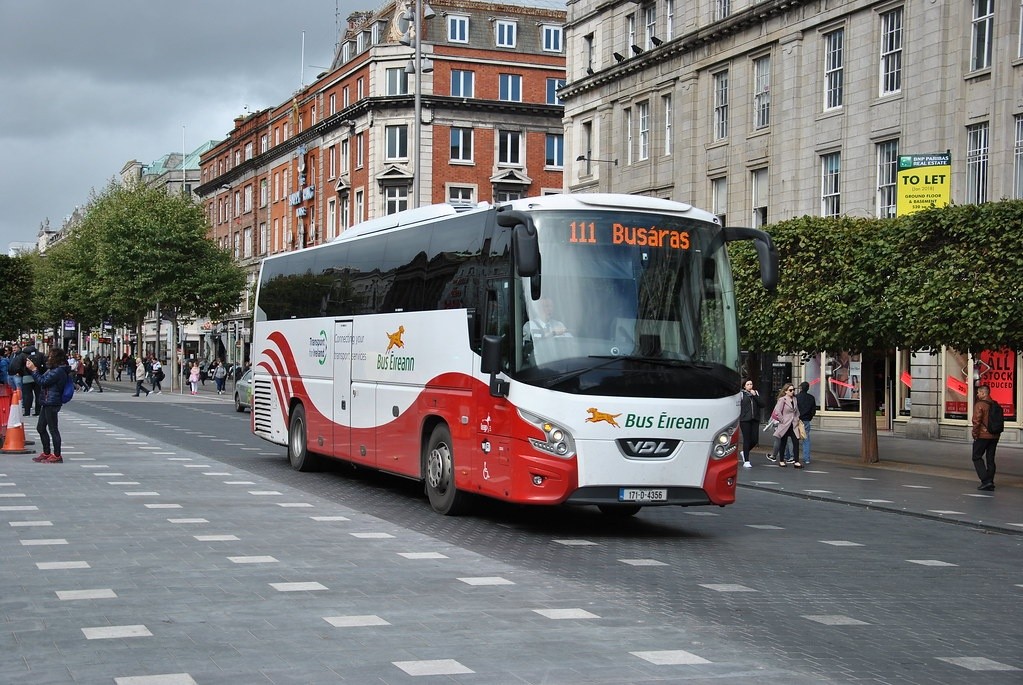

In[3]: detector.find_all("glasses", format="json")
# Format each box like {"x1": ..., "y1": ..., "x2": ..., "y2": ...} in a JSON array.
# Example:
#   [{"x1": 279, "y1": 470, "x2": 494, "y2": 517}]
[
  {"x1": 745, "y1": 384, "x2": 752, "y2": 386},
  {"x1": 787, "y1": 388, "x2": 795, "y2": 392}
]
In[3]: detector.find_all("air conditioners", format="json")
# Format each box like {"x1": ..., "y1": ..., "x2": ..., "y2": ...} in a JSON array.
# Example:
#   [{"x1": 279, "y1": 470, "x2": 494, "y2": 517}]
[{"x1": 296, "y1": 206, "x2": 306, "y2": 219}]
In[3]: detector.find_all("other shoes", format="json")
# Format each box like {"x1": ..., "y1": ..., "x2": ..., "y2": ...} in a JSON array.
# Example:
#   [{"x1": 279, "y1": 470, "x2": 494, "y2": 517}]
[
  {"x1": 192, "y1": 391, "x2": 196, "y2": 395},
  {"x1": 988, "y1": 486, "x2": 994, "y2": 490},
  {"x1": 794, "y1": 463, "x2": 804, "y2": 469},
  {"x1": 740, "y1": 450, "x2": 745, "y2": 462},
  {"x1": 786, "y1": 460, "x2": 795, "y2": 464},
  {"x1": 804, "y1": 461, "x2": 810, "y2": 464},
  {"x1": 217, "y1": 389, "x2": 225, "y2": 395},
  {"x1": 977, "y1": 481, "x2": 992, "y2": 489},
  {"x1": 766, "y1": 453, "x2": 776, "y2": 462},
  {"x1": 779, "y1": 462, "x2": 787, "y2": 467},
  {"x1": 7, "y1": 376, "x2": 163, "y2": 417},
  {"x1": 784, "y1": 458, "x2": 788, "y2": 460},
  {"x1": 743, "y1": 461, "x2": 752, "y2": 468}
]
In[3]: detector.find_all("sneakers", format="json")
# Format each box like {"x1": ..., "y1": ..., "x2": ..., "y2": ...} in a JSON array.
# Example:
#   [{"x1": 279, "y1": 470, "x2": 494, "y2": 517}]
[
  {"x1": 40, "y1": 454, "x2": 63, "y2": 463},
  {"x1": 32, "y1": 452, "x2": 52, "y2": 462}
]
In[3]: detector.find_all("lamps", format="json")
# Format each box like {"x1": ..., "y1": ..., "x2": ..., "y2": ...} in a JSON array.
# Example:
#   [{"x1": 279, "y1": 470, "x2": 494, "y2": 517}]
[{"x1": 219, "y1": 180, "x2": 232, "y2": 190}]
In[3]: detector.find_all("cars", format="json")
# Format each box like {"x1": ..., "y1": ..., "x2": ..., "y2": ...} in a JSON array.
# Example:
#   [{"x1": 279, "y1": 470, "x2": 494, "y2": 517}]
[{"x1": 234, "y1": 369, "x2": 250, "y2": 412}]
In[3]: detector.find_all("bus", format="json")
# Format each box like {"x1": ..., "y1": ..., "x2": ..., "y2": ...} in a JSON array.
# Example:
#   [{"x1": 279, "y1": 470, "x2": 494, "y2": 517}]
[{"x1": 250, "y1": 193, "x2": 780, "y2": 516}]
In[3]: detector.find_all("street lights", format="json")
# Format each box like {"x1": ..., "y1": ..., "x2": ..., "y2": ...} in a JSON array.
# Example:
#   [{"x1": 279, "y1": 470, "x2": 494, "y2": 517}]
[{"x1": 399, "y1": 1, "x2": 437, "y2": 208}]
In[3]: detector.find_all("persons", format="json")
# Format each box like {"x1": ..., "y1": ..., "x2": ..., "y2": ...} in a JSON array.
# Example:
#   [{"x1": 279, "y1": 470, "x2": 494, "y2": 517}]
[
  {"x1": 26, "y1": 348, "x2": 71, "y2": 464},
  {"x1": 178, "y1": 358, "x2": 249, "y2": 394},
  {"x1": 739, "y1": 378, "x2": 766, "y2": 468},
  {"x1": 961, "y1": 353, "x2": 990, "y2": 398},
  {"x1": 523, "y1": 299, "x2": 573, "y2": 358},
  {"x1": 972, "y1": 386, "x2": 1001, "y2": 491},
  {"x1": 0, "y1": 338, "x2": 166, "y2": 416},
  {"x1": 766, "y1": 381, "x2": 816, "y2": 468},
  {"x1": 837, "y1": 348, "x2": 861, "y2": 399}
]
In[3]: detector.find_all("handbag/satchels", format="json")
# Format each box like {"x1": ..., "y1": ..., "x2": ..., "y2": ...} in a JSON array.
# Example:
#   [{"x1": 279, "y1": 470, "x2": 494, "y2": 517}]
[
  {"x1": 186, "y1": 380, "x2": 190, "y2": 385},
  {"x1": 157, "y1": 367, "x2": 165, "y2": 381},
  {"x1": 798, "y1": 420, "x2": 807, "y2": 440}
]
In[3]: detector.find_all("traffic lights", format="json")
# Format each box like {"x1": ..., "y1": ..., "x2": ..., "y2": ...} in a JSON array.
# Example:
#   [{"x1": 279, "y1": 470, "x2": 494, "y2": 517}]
[{"x1": 176, "y1": 326, "x2": 180, "y2": 344}]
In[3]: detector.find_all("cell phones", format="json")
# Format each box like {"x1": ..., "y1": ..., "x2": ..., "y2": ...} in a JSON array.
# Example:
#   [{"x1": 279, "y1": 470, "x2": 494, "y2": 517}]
[{"x1": 27, "y1": 358, "x2": 32, "y2": 363}]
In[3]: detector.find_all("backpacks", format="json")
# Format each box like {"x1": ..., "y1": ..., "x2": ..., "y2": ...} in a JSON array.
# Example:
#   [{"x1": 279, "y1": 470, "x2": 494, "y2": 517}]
[
  {"x1": 977, "y1": 399, "x2": 1004, "y2": 434},
  {"x1": 57, "y1": 366, "x2": 74, "y2": 403},
  {"x1": 23, "y1": 350, "x2": 44, "y2": 375}
]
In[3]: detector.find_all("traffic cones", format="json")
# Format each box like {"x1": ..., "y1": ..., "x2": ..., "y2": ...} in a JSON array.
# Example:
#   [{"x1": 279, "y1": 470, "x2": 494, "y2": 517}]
[{"x1": 0, "y1": 387, "x2": 36, "y2": 454}]
